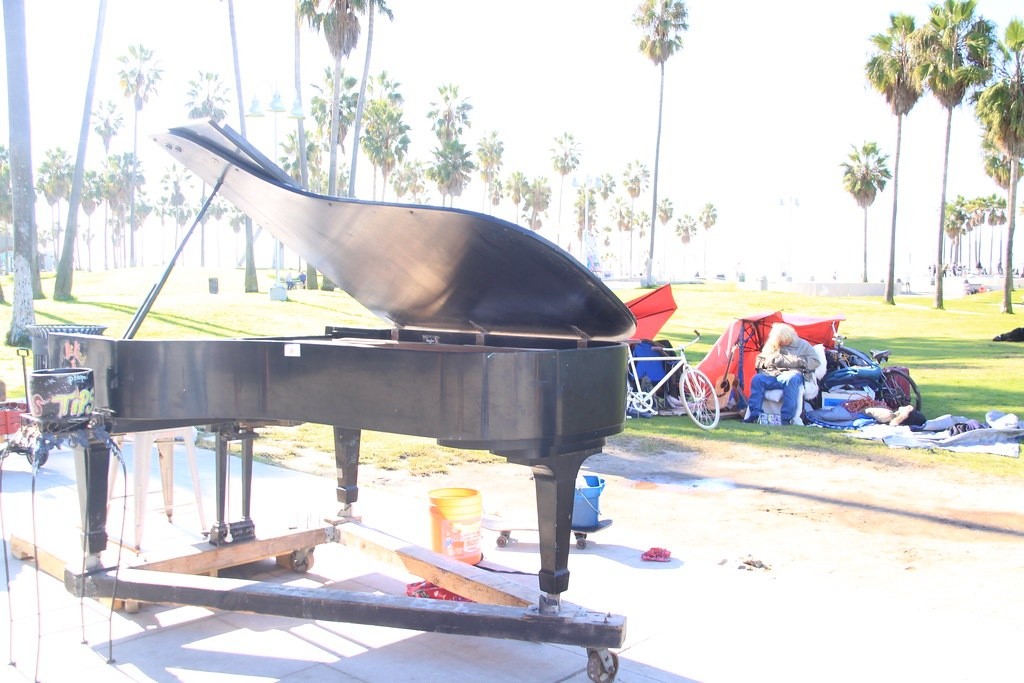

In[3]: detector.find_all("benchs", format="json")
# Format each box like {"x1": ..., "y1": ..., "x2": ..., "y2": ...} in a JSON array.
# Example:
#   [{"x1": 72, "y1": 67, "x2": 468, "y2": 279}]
[{"x1": 22, "y1": 415, "x2": 303, "y2": 566}]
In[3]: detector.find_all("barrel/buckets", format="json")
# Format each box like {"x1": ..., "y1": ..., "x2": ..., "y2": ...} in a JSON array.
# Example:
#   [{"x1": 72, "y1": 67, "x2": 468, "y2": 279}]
[
  {"x1": 426, "y1": 486, "x2": 483, "y2": 565},
  {"x1": 569, "y1": 474, "x2": 606, "y2": 528}
]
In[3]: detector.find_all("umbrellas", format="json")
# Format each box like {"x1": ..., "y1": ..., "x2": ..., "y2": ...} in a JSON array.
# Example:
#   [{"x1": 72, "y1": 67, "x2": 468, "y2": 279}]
[
  {"x1": 623, "y1": 283, "x2": 678, "y2": 398},
  {"x1": 684, "y1": 307, "x2": 847, "y2": 410}
]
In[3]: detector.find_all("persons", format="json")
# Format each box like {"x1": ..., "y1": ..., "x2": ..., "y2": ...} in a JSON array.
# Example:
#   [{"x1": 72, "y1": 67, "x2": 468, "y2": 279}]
[
  {"x1": 298, "y1": 270, "x2": 306, "y2": 289},
  {"x1": 284, "y1": 268, "x2": 295, "y2": 290},
  {"x1": 927, "y1": 261, "x2": 1024, "y2": 296},
  {"x1": 743, "y1": 321, "x2": 822, "y2": 425}
]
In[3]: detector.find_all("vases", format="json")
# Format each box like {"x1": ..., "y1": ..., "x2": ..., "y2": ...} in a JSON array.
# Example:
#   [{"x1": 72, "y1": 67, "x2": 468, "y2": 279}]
[{"x1": 30, "y1": 367, "x2": 94, "y2": 434}]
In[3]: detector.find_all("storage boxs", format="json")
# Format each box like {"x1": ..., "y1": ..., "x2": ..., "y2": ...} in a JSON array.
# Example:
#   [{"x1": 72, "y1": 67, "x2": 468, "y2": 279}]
[
  {"x1": 0, "y1": 402, "x2": 29, "y2": 434},
  {"x1": 571, "y1": 474, "x2": 606, "y2": 528}
]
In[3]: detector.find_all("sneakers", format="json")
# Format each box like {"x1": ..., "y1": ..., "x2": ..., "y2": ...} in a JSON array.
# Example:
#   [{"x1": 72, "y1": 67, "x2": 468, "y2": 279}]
[
  {"x1": 780, "y1": 419, "x2": 793, "y2": 425},
  {"x1": 742, "y1": 415, "x2": 761, "y2": 424}
]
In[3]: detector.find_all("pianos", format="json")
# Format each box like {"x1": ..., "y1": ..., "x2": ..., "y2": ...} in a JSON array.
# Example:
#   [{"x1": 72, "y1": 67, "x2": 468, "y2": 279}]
[{"x1": 48, "y1": 120, "x2": 638, "y2": 682}]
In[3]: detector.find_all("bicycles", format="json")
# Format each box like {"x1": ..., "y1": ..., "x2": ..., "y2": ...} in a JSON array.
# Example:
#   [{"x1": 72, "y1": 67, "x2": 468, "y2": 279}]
[
  {"x1": 831, "y1": 320, "x2": 921, "y2": 412},
  {"x1": 622, "y1": 329, "x2": 721, "y2": 430}
]
